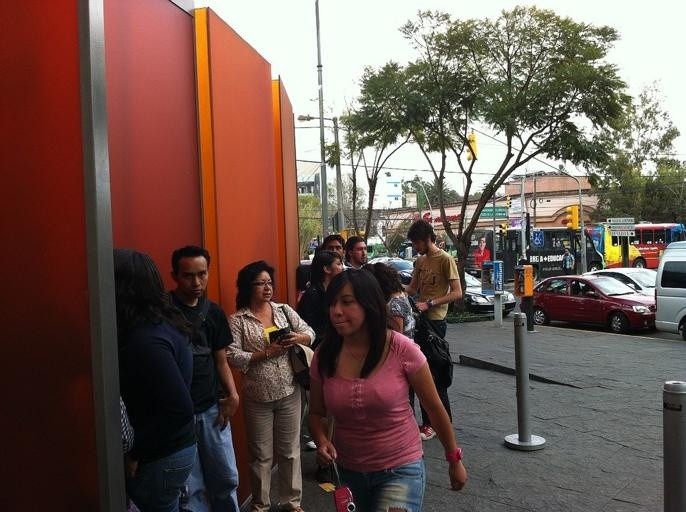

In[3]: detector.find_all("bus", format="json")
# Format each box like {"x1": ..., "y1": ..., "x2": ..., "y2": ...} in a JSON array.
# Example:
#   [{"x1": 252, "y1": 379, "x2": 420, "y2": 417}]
[
  {"x1": 463, "y1": 227, "x2": 604, "y2": 283},
  {"x1": 585, "y1": 221, "x2": 686, "y2": 270}
]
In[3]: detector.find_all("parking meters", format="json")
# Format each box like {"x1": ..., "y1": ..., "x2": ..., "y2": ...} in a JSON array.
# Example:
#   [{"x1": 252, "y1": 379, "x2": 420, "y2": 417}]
[{"x1": 511, "y1": 263, "x2": 535, "y2": 332}]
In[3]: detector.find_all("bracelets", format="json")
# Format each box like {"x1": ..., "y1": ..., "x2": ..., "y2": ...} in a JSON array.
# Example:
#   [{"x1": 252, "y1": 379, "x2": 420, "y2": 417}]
[{"x1": 266, "y1": 350, "x2": 269, "y2": 360}]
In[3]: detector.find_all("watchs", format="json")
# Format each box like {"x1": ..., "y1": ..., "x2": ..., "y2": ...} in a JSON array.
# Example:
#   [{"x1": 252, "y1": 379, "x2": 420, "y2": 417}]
[{"x1": 446, "y1": 448, "x2": 464, "y2": 462}]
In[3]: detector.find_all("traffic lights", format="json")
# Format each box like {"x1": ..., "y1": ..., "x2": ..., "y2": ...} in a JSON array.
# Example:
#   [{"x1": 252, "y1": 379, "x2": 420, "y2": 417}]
[
  {"x1": 505, "y1": 196, "x2": 512, "y2": 209},
  {"x1": 465, "y1": 135, "x2": 478, "y2": 160},
  {"x1": 566, "y1": 205, "x2": 579, "y2": 230},
  {"x1": 499, "y1": 223, "x2": 508, "y2": 237},
  {"x1": 355, "y1": 230, "x2": 365, "y2": 239}
]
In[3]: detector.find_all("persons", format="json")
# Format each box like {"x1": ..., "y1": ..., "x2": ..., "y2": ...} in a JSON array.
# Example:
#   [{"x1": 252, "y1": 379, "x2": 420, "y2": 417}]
[
  {"x1": 113, "y1": 245, "x2": 239, "y2": 511},
  {"x1": 563, "y1": 248, "x2": 576, "y2": 274},
  {"x1": 403, "y1": 221, "x2": 462, "y2": 440},
  {"x1": 309, "y1": 268, "x2": 467, "y2": 512},
  {"x1": 471, "y1": 236, "x2": 491, "y2": 268},
  {"x1": 226, "y1": 260, "x2": 315, "y2": 511},
  {"x1": 297, "y1": 236, "x2": 416, "y2": 451}
]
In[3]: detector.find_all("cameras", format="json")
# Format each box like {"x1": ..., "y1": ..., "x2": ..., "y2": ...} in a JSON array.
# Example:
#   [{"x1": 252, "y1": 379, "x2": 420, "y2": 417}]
[{"x1": 335, "y1": 485, "x2": 356, "y2": 512}]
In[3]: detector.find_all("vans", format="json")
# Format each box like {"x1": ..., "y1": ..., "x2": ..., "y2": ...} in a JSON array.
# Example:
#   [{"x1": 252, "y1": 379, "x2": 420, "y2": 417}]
[{"x1": 654, "y1": 242, "x2": 685, "y2": 345}]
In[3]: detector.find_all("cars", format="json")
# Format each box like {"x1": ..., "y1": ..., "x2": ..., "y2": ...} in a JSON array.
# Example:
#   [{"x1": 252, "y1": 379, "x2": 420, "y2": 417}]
[
  {"x1": 450, "y1": 271, "x2": 516, "y2": 316},
  {"x1": 521, "y1": 273, "x2": 656, "y2": 334},
  {"x1": 571, "y1": 268, "x2": 658, "y2": 295},
  {"x1": 378, "y1": 259, "x2": 414, "y2": 286}
]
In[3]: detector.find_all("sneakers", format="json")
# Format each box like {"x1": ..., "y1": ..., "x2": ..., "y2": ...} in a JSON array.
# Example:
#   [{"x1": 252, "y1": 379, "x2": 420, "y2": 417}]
[{"x1": 418, "y1": 423, "x2": 437, "y2": 441}]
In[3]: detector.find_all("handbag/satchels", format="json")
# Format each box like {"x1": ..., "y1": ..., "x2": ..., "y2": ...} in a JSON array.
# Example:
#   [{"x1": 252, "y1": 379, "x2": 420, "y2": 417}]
[
  {"x1": 420, "y1": 324, "x2": 452, "y2": 388},
  {"x1": 288, "y1": 344, "x2": 314, "y2": 391}
]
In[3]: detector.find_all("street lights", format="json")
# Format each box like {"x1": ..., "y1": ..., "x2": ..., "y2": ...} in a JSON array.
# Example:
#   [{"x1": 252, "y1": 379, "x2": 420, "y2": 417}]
[
  {"x1": 402, "y1": 181, "x2": 435, "y2": 231},
  {"x1": 297, "y1": 114, "x2": 345, "y2": 243}
]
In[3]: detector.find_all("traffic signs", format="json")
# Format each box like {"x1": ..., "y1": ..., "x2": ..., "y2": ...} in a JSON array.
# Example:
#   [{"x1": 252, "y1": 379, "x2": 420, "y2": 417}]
[
  {"x1": 606, "y1": 217, "x2": 634, "y2": 223},
  {"x1": 607, "y1": 225, "x2": 635, "y2": 230},
  {"x1": 610, "y1": 231, "x2": 635, "y2": 236}
]
